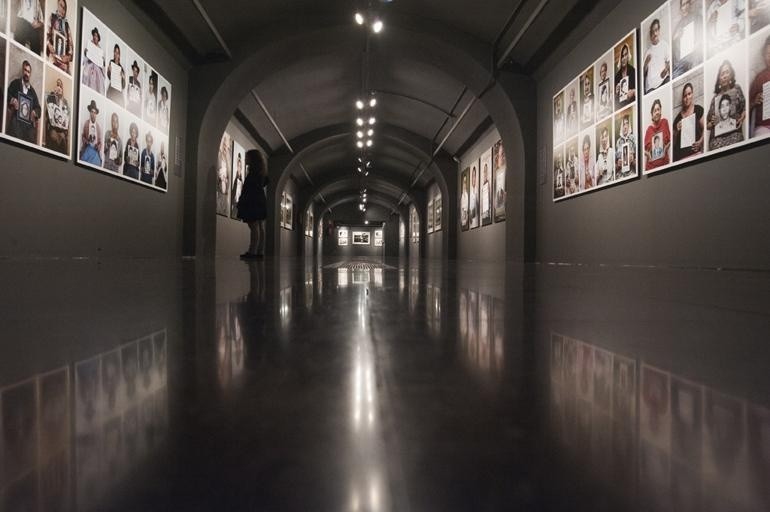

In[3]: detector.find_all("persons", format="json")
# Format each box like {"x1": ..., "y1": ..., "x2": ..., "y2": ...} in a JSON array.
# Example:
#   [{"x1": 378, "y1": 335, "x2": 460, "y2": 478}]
[
  {"x1": 5, "y1": 0, "x2": 170, "y2": 190},
  {"x1": 237, "y1": 259, "x2": 273, "y2": 370},
  {"x1": 236, "y1": 149, "x2": 271, "y2": 259},
  {"x1": 457, "y1": 137, "x2": 506, "y2": 232},
  {"x1": 553, "y1": 0, "x2": 769, "y2": 202},
  {"x1": 217, "y1": 135, "x2": 244, "y2": 221}
]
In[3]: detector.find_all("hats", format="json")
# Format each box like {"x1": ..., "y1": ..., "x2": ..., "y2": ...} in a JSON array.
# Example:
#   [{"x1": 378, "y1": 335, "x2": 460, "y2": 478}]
[
  {"x1": 92, "y1": 27, "x2": 100, "y2": 41},
  {"x1": 132, "y1": 61, "x2": 139, "y2": 73},
  {"x1": 88, "y1": 101, "x2": 99, "y2": 114}
]
